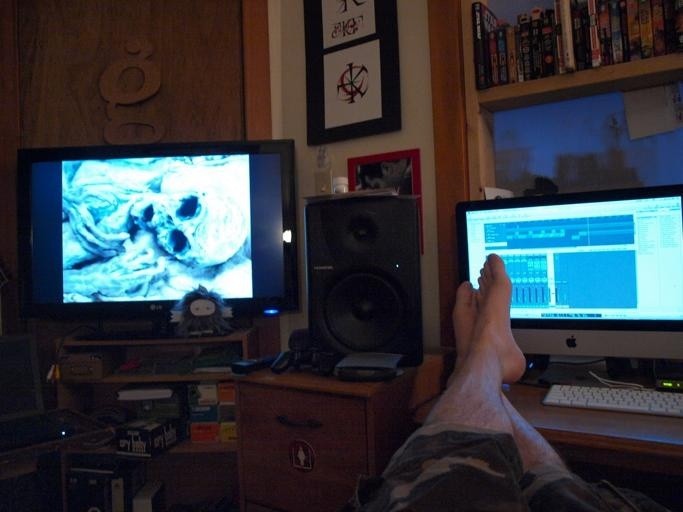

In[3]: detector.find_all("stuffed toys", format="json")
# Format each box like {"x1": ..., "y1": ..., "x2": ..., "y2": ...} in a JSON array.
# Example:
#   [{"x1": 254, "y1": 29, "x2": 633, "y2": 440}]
[{"x1": 167, "y1": 287, "x2": 235, "y2": 337}]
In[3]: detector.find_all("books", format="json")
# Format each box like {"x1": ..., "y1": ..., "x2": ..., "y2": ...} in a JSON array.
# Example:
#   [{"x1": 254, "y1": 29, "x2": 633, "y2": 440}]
[
  {"x1": 115, "y1": 383, "x2": 172, "y2": 401},
  {"x1": 470, "y1": 0, "x2": 682, "y2": 91}
]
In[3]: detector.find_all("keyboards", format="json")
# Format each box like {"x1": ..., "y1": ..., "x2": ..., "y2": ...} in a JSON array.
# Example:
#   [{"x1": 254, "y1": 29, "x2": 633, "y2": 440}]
[{"x1": 542, "y1": 381, "x2": 683, "y2": 418}]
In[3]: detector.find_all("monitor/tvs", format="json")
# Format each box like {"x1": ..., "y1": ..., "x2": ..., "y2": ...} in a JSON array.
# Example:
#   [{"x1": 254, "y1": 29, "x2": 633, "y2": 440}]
[
  {"x1": 455, "y1": 184, "x2": 683, "y2": 391},
  {"x1": 13, "y1": 137, "x2": 301, "y2": 336}
]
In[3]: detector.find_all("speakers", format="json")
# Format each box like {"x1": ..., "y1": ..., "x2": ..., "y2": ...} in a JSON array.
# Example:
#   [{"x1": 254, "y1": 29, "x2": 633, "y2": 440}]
[{"x1": 302, "y1": 194, "x2": 423, "y2": 370}]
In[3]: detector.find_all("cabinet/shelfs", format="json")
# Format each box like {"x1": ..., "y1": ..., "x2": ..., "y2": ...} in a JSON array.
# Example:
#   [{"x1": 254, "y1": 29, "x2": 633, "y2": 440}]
[
  {"x1": 235, "y1": 352, "x2": 447, "y2": 512},
  {"x1": 52, "y1": 312, "x2": 283, "y2": 512}
]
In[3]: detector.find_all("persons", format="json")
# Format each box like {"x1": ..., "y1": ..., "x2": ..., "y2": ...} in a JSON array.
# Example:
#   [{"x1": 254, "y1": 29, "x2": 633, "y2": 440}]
[
  {"x1": 347, "y1": 254, "x2": 677, "y2": 511},
  {"x1": 357, "y1": 158, "x2": 411, "y2": 195}
]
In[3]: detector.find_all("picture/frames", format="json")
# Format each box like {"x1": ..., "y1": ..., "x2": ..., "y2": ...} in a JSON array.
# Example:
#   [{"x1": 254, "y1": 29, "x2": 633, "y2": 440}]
[{"x1": 301, "y1": 0, "x2": 425, "y2": 256}]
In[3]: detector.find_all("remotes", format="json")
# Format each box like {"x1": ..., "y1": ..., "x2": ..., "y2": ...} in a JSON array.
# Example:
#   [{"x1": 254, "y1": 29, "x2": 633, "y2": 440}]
[{"x1": 231, "y1": 353, "x2": 271, "y2": 375}]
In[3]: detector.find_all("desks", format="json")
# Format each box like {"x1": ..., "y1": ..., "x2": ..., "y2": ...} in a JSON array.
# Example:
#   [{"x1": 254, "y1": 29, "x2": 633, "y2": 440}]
[{"x1": 414, "y1": 368, "x2": 683, "y2": 481}]
